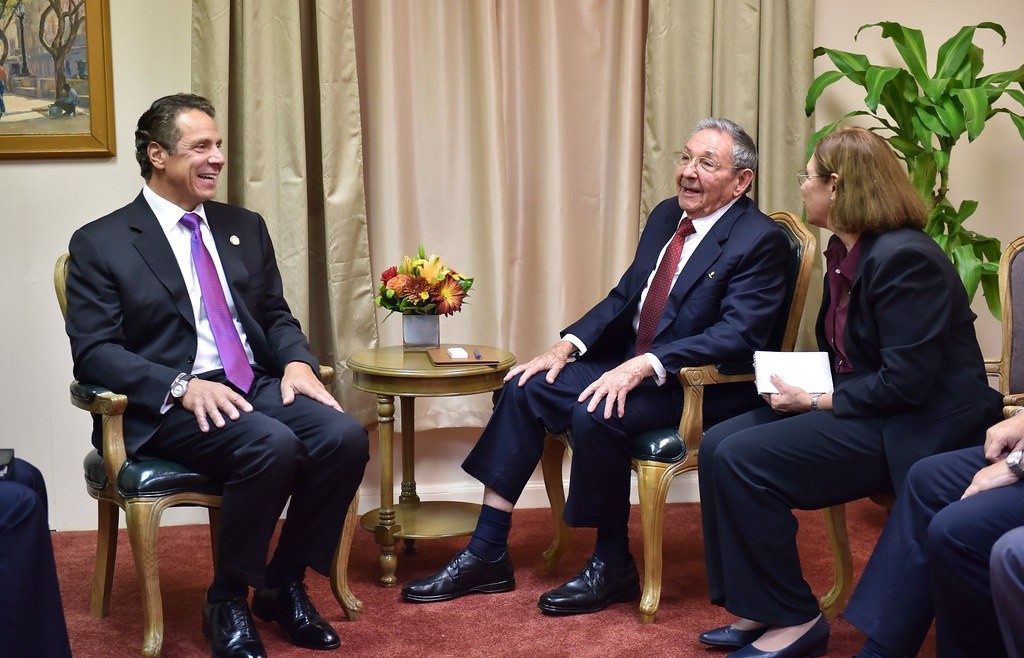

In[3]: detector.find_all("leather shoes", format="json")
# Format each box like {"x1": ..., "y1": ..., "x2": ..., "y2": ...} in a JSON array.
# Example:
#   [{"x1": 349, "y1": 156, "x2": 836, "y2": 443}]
[
  {"x1": 537, "y1": 554, "x2": 641, "y2": 614},
  {"x1": 202, "y1": 592, "x2": 267, "y2": 658},
  {"x1": 251, "y1": 581, "x2": 341, "y2": 650},
  {"x1": 401, "y1": 547, "x2": 515, "y2": 601},
  {"x1": 726, "y1": 612, "x2": 831, "y2": 658},
  {"x1": 698, "y1": 624, "x2": 772, "y2": 646}
]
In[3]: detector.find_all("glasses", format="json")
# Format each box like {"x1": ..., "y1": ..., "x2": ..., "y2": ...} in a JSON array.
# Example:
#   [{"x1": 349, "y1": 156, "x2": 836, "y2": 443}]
[
  {"x1": 797, "y1": 169, "x2": 821, "y2": 186},
  {"x1": 673, "y1": 151, "x2": 746, "y2": 174}
]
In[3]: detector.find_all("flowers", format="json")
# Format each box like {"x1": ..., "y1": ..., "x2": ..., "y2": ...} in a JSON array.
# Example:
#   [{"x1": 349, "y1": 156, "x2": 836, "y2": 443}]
[{"x1": 375, "y1": 243, "x2": 474, "y2": 325}]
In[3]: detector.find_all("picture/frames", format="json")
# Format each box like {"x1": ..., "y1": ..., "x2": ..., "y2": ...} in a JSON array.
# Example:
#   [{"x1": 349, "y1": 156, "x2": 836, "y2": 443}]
[{"x1": 0, "y1": 0, "x2": 117, "y2": 158}]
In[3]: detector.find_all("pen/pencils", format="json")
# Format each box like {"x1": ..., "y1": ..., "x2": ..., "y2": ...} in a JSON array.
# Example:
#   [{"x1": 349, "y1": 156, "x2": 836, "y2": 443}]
[{"x1": 474, "y1": 351, "x2": 480, "y2": 359}]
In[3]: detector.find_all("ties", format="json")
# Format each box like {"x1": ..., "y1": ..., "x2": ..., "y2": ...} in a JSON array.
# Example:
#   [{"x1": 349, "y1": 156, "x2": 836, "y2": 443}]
[
  {"x1": 635, "y1": 217, "x2": 697, "y2": 355},
  {"x1": 179, "y1": 214, "x2": 255, "y2": 393}
]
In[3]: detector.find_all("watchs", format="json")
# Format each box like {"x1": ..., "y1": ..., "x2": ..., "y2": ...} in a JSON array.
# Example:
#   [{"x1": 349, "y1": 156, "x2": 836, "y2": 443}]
[
  {"x1": 810, "y1": 392, "x2": 826, "y2": 412},
  {"x1": 1005, "y1": 449, "x2": 1024, "y2": 479},
  {"x1": 169, "y1": 374, "x2": 198, "y2": 407}
]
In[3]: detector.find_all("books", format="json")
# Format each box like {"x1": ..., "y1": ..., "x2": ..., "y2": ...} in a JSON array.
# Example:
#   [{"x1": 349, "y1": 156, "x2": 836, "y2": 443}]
[
  {"x1": 753, "y1": 351, "x2": 834, "y2": 397},
  {"x1": 427, "y1": 346, "x2": 500, "y2": 367}
]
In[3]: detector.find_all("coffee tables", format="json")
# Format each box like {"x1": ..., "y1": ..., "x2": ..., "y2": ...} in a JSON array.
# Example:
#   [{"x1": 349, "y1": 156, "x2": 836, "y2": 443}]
[{"x1": 346, "y1": 345, "x2": 518, "y2": 587}]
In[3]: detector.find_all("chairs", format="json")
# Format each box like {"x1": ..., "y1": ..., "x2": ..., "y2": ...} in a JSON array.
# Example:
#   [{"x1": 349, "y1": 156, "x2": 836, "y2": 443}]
[
  {"x1": 820, "y1": 236, "x2": 1024, "y2": 617},
  {"x1": 542, "y1": 210, "x2": 816, "y2": 624},
  {"x1": 55, "y1": 253, "x2": 361, "y2": 658}
]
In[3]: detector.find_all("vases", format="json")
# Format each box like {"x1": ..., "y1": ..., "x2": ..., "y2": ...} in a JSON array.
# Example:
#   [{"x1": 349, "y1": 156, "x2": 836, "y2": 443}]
[{"x1": 402, "y1": 314, "x2": 440, "y2": 353}]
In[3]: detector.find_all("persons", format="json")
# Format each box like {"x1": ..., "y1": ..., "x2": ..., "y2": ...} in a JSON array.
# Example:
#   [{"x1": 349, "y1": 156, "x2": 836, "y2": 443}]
[
  {"x1": 0, "y1": 448, "x2": 72, "y2": 658},
  {"x1": 839, "y1": 405, "x2": 1024, "y2": 658},
  {"x1": 698, "y1": 126, "x2": 993, "y2": 658},
  {"x1": 65, "y1": 93, "x2": 373, "y2": 658},
  {"x1": 400, "y1": 119, "x2": 798, "y2": 616}
]
